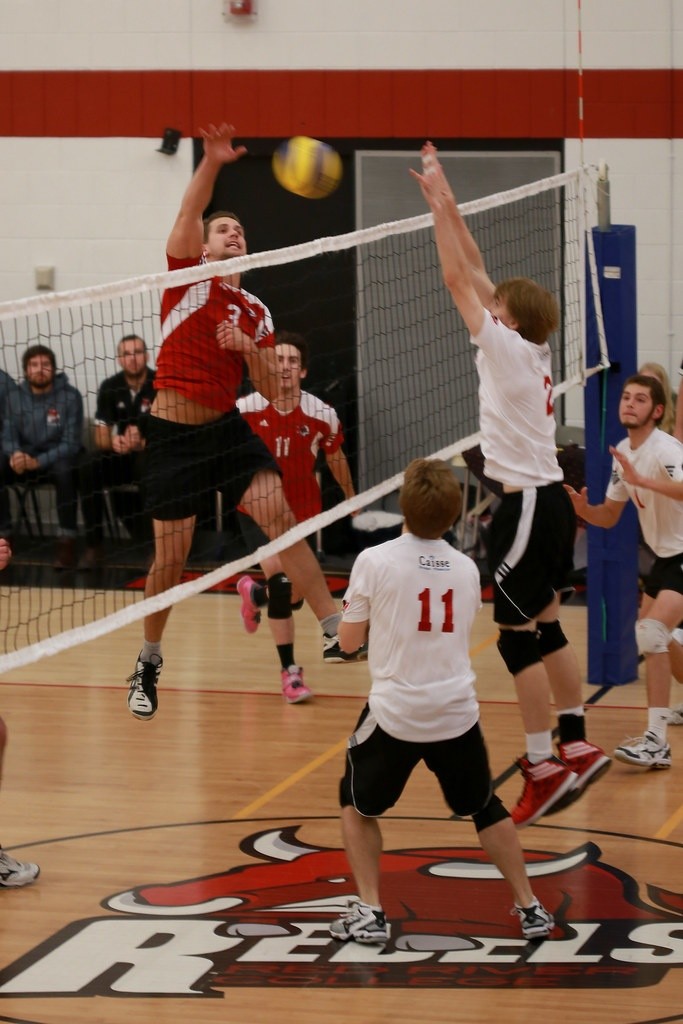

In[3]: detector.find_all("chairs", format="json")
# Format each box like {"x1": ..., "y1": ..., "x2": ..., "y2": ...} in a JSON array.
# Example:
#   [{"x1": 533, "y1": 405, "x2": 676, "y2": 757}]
[{"x1": 0, "y1": 480, "x2": 209, "y2": 549}]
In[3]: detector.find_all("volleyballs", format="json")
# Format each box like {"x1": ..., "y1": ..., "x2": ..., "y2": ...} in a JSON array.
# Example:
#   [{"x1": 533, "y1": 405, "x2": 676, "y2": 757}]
[{"x1": 273, "y1": 135, "x2": 344, "y2": 199}]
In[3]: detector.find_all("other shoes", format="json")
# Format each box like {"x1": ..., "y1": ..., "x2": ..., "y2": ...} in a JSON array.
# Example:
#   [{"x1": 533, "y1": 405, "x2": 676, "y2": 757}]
[
  {"x1": 128, "y1": 542, "x2": 154, "y2": 568},
  {"x1": 50, "y1": 537, "x2": 82, "y2": 569},
  {"x1": 0, "y1": 522, "x2": 13, "y2": 537},
  {"x1": 77, "y1": 541, "x2": 111, "y2": 575}
]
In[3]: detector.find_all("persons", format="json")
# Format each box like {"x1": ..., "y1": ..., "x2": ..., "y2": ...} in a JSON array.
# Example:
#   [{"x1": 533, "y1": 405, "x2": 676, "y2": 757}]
[
  {"x1": 77, "y1": 335, "x2": 157, "y2": 570},
  {"x1": 127, "y1": 124, "x2": 368, "y2": 727},
  {"x1": 410, "y1": 138, "x2": 611, "y2": 830},
  {"x1": 237, "y1": 330, "x2": 361, "y2": 705},
  {"x1": 0, "y1": 540, "x2": 41, "y2": 889},
  {"x1": 329, "y1": 461, "x2": 556, "y2": 943},
  {"x1": 0, "y1": 346, "x2": 82, "y2": 549},
  {"x1": 563, "y1": 359, "x2": 683, "y2": 769}
]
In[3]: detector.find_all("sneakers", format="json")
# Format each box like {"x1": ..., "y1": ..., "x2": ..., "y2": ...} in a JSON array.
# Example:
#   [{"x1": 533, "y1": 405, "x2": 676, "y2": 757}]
[
  {"x1": 0, "y1": 852, "x2": 40, "y2": 887},
  {"x1": 281, "y1": 665, "x2": 314, "y2": 704},
  {"x1": 329, "y1": 899, "x2": 387, "y2": 943},
  {"x1": 668, "y1": 703, "x2": 683, "y2": 725},
  {"x1": 514, "y1": 895, "x2": 556, "y2": 939},
  {"x1": 125, "y1": 648, "x2": 163, "y2": 721},
  {"x1": 322, "y1": 632, "x2": 368, "y2": 664},
  {"x1": 509, "y1": 755, "x2": 578, "y2": 830},
  {"x1": 236, "y1": 576, "x2": 263, "y2": 634},
  {"x1": 542, "y1": 740, "x2": 612, "y2": 817},
  {"x1": 613, "y1": 730, "x2": 672, "y2": 768}
]
